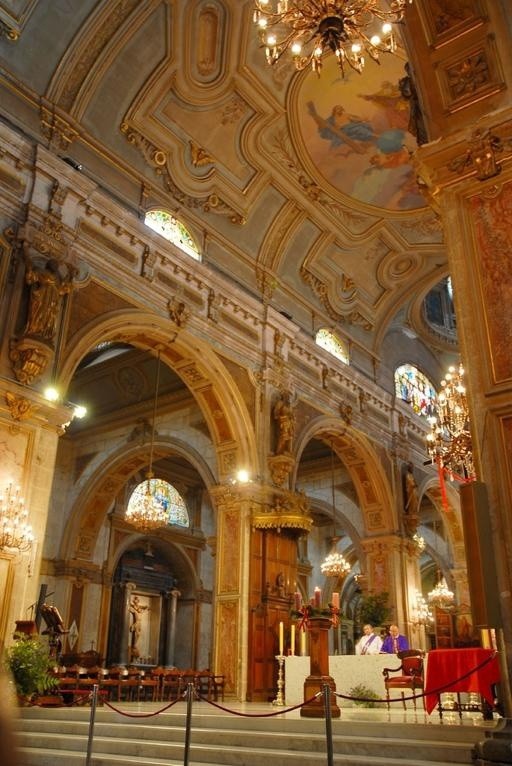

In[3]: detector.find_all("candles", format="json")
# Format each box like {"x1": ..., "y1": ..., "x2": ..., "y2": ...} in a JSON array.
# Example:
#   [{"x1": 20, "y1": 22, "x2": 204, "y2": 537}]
[
  {"x1": 299, "y1": 628, "x2": 306, "y2": 656},
  {"x1": 311, "y1": 597, "x2": 315, "y2": 609},
  {"x1": 314, "y1": 586, "x2": 321, "y2": 609},
  {"x1": 291, "y1": 623, "x2": 295, "y2": 655},
  {"x1": 294, "y1": 592, "x2": 301, "y2": 611},
  {"x1": 279, "y1": 621, "x2": 284, "y2": 656},
  {"x1": 332, "y1": 592, "x2": 340, "y2": 609}
]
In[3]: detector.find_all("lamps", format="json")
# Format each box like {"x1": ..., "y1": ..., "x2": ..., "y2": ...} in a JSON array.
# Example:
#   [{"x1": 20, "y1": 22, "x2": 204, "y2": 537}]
[
  {"x1": 124, "y1": 344, "x2": 190, "y2": 534},
  {"x1": 428, "y1": 503, "x2": 455, "y2": 609},
  {"x1": 39, "y1": 384, "x2": 88, "y2": 419},
  {"x1": 0, "y1": 476, "x2": 36, "y2": 577},
  {"x1": 320, "y1": 438, "x2": 351, "y2": 579},
  {"x1": 426, "y1": 353, "x2": 478, "y2": 483},
  {"x1": 251, "y1": 0, "x2": 413, "y2": 79}
]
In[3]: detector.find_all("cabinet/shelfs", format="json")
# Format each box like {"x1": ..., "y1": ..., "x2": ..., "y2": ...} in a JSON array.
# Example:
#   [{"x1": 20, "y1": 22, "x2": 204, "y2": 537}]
[{"x1": 432, "y1": 606, "x2": 454, "y2": 649}]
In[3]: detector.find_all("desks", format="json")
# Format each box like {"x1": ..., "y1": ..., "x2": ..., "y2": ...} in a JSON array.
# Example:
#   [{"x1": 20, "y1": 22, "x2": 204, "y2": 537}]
[{"x1": 424, "y1": 648, "x2": 501, "y2": 719}]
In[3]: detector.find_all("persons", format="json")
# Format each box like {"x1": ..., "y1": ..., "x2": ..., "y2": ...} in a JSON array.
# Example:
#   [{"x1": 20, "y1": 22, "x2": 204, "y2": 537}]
[
  {"x1": 405, "y1": 460, "x2": 418, "y2": 514},
  {"x1": 273, "y1": 389, "x2": 296, "y2": 455},
  {"x1": 129, "y1": 597, "x2": 151, "y2": 650},
  {"x1": 318, "y1": 80, "x2": 421, "y2": 171},
  {"x1": 379, "y1": 626, "x2": 410, "y2": 654},
  {"x1": 354, "y1": 624, "x2": 383, "y2": 655},
  {"x1": 130, "y1": 606, "x2": 150, "y2": 650},
  {"x1": 24, "y1": 258, "x2": 74, "y2": 343}
]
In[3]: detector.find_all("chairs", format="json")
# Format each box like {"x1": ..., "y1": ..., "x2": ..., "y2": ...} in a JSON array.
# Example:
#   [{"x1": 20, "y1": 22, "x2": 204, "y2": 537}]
[
  {"x1": 47, "y1": 656, "x2": 225, "y2": 707},
  {"x1": 382, "y1": 650, "x2": 424, "y2": 709}
]
